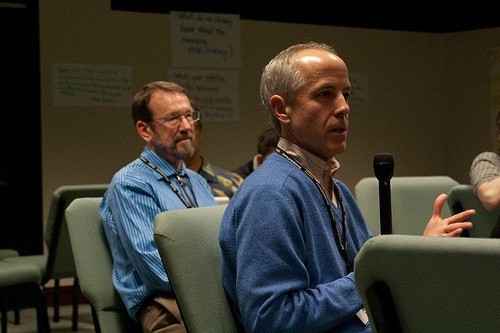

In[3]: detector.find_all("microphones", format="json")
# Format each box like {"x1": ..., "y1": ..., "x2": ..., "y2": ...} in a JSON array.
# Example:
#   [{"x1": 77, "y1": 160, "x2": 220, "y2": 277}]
[{"x1": 373, "y1": 152, "x2": 395, "y2": 235}]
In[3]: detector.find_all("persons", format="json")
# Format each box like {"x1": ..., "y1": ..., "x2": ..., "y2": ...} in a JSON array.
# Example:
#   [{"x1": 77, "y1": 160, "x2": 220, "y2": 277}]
[
  {"x1": 233, "y1": 127, "x2": 280, "y2": 181},
  {"x1": 218, "y1": 42, "x2": 475, "y2": 333},
  {"x1": 468, "y1": 110, "x2": 500, "y2": 238},
  {"x1": 184, "y1": 101, "x2": 246, "y2": 199},
  {"x1": 98, "y1": 82, "x2": 218, "y2": 333}
]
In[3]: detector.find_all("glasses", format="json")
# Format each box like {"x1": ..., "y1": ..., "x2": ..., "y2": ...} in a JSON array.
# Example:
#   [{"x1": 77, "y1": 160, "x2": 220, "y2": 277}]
[{"x1": 150, "y1": 111, "x2": 200, "y2": 127}]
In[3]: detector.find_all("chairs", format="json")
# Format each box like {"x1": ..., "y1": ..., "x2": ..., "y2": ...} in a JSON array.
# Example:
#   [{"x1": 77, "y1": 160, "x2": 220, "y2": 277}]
[{"x1": 0, "y1": 176, "x2": 500, "y2": 333}]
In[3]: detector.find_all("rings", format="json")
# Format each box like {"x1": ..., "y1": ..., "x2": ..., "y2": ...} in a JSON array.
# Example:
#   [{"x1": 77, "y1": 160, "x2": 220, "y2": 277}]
[{"x1": 442, "y1": 233, "x2": 447, "y2": 238}]
